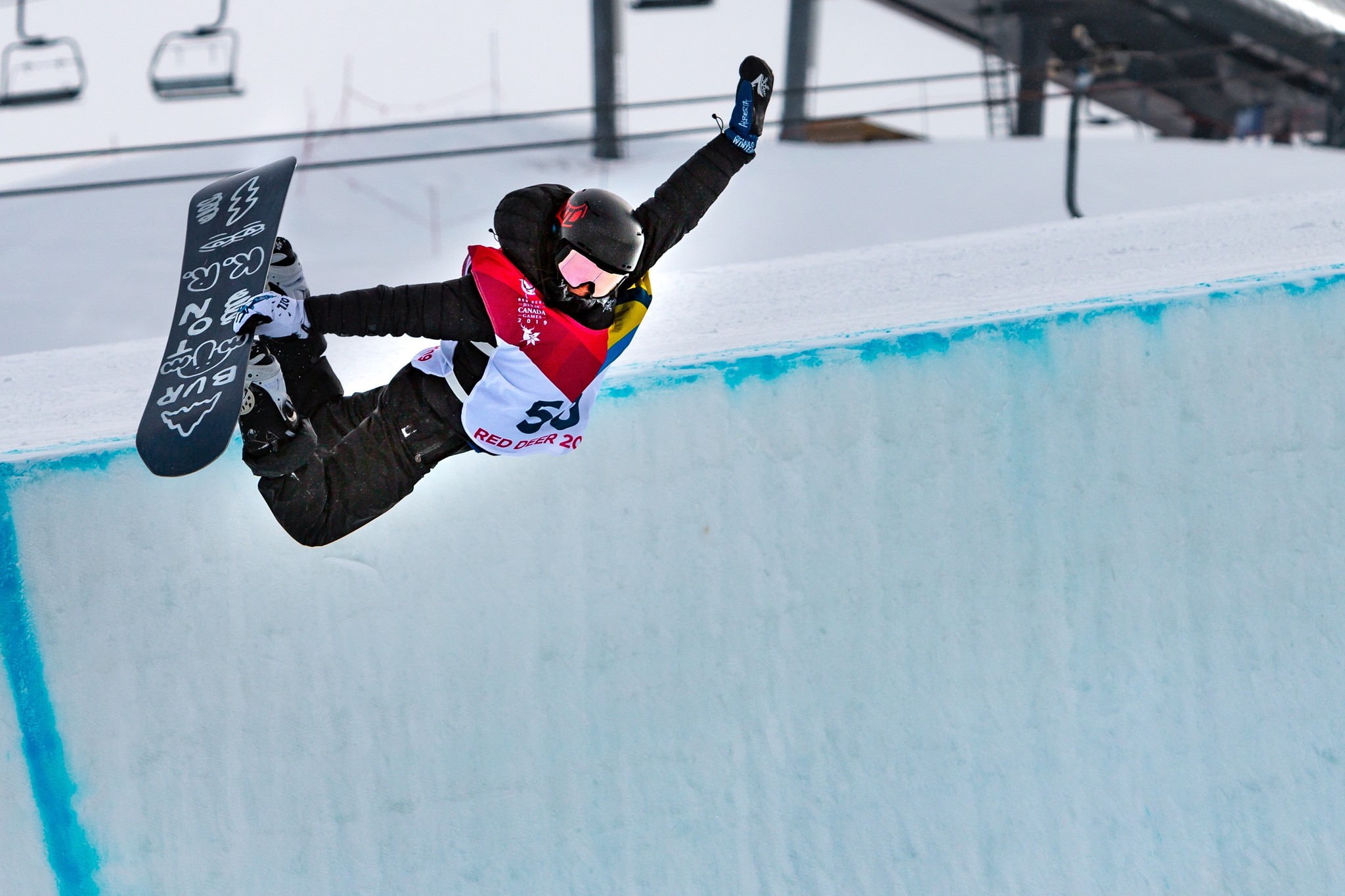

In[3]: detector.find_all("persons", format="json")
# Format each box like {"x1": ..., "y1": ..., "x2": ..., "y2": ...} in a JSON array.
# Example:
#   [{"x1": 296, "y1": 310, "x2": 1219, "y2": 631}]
[{"x1": 232, "y1": 56, "x2": 775, "y2": 545}]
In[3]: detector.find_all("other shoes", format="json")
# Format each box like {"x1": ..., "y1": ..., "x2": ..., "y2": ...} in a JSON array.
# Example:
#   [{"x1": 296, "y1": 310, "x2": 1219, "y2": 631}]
[
  {"x1": 235, "y1": 344, "x2": 299, "y2": 450},
  {"x1": 264, "y1": 236, "x2": 309, "y2": 299}
]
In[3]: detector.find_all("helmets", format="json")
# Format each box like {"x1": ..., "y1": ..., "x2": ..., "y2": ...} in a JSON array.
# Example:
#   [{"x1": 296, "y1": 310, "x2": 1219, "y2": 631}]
[{"x1": 556, "y1": 190, "x2": 644, "y2": 272}]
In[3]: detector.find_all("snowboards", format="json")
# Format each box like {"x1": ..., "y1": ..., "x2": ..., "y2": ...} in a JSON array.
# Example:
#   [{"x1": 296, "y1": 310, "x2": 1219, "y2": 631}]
[{"x1": 133, "y1": 154, "x2": 299, "y2": 479}]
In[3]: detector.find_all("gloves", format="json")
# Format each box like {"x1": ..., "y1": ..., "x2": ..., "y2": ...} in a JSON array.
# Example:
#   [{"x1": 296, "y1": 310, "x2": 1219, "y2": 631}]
[
  {"x1": 232, "y1": 290, "x2": 310, "y2": 340},
  {"x1": 723, "y1": 56, "x2": 775, "y2": 154}
]
